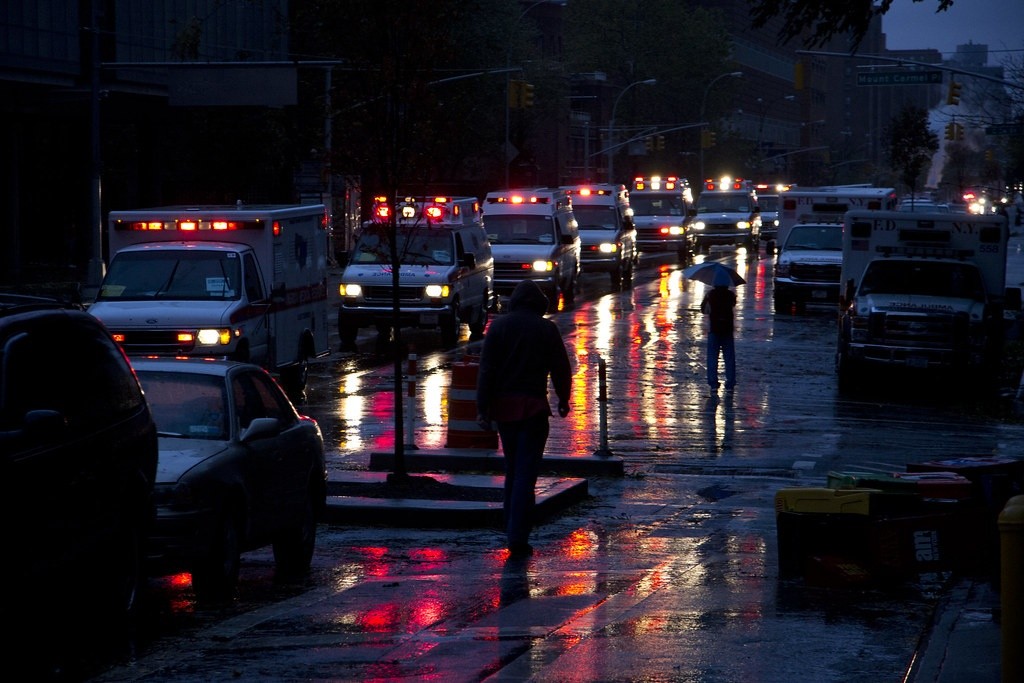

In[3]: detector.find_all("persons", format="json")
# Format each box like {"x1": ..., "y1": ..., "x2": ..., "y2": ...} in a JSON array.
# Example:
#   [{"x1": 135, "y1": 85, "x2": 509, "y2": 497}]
[
  {"x1": 475, "y1": 281, "x2": 572, "y2": 555},
  {"x1": 700, "y1": 286, "x2": 737, "y2": 390},
  {"x1": 998, "y1": 207, "x2": 1008, "y2": 218}
]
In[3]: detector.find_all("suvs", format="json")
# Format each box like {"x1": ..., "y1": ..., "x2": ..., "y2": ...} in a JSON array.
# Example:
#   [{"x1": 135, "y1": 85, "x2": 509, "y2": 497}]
[
  {"x1": 479, "y1": 185, "x2": 583, "y2": 315},
  {"x1": 626, "y1": 173, "x2": 694, "y2": 266},
  {"x1": 0, "y1": 291, "x2": 160, "y2": 650},
  {"x1": 693, "y1": 171, "x2": 763, "y2": 256},
  {"x1": 336, "y1": 193, "x2": 496, "y2": 347},
  {"x1": 556, "y1": 182, "x2": 639, "y2": 291}
]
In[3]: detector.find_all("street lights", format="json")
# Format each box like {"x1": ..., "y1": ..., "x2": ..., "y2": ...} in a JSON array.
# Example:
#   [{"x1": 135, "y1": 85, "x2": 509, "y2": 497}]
[
  {"x1": 699, "y1": 70, "x2": 743, "y2": 182},
  {"x1": 758, "y1": 93, "x2": 795, "y2": 171},
  {"x1": 607, "y1": 78, "x2": 658, "y2": 184},
  {"x1": 787, "y1": 119, "x2": 826, "y2": 181},
  {"x1": 503, "y1": 0, "x2": 568, "y2": 189}
]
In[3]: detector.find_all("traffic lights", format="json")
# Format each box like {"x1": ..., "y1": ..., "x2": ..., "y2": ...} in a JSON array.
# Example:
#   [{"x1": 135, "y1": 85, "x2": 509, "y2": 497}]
[
  {"x1": 957, "y1": 124, "x2": 965, "y2": 142},
  {"x1": 946, "y1": 80, "x2": 962, "y2": 105},
  {"x1": 984, "y1": 150, "x2": 993, "y2": 161},
  {"x1": 944, "y1": 123, "x2": 954, "y2": 141}
]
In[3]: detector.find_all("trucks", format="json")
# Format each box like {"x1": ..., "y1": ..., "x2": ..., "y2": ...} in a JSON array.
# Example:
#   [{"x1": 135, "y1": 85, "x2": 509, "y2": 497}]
[
  {"x1": 82, "y1": 201, "x2": 332, "y2": 393},
  {"x1": 753, "y1": 178, "x2": 972, "y2": 320},
  {"x1": 832, "y1": 209, "x2": 1010, "y2": 392}
]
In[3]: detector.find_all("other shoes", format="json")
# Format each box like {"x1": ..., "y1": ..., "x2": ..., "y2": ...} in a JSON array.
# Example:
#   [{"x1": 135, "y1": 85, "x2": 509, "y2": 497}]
[
  {"x1": 725, "y1": 386, "x2": 735, "y2": 392},
  {"x1": 711, "y1": 386, "x2": 718, "y2": 392}
]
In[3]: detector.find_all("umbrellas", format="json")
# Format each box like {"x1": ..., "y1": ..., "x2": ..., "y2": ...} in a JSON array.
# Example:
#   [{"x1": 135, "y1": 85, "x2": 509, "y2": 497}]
[{"x1": 682, "y1": 261, "x2": 746, "y2": 287}]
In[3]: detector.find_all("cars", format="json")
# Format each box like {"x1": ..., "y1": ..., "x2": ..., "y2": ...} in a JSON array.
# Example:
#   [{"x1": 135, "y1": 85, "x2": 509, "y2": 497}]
[{"x1": 122, "y1": 354, "x2": 327, "y2": 598}]
